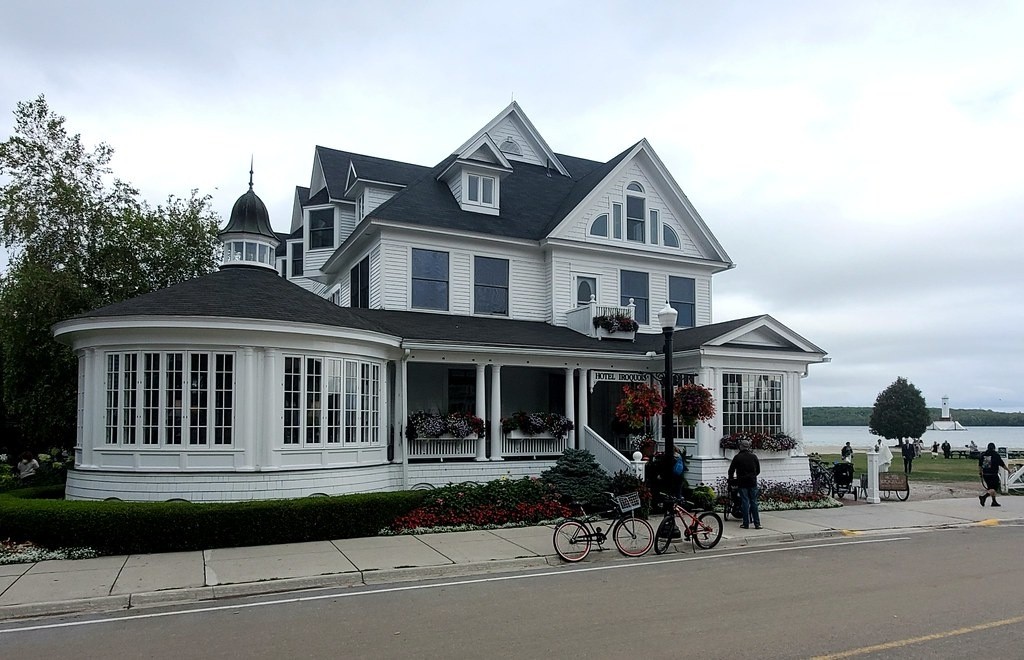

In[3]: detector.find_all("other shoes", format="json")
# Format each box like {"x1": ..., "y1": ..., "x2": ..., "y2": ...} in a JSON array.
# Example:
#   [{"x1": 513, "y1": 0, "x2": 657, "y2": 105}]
[
  {"x1": 979, "y1": 496, "x2": 986, "y2": 506},
  {"x1": 991, "y1": 502, "x2": 1001, "y2": 507},
  {"x1": 755, "y1": 525, "x2": 763, "y2": 529},
  {"x1": 740, "y1": 524, "x2": 749, "y2": 528}
]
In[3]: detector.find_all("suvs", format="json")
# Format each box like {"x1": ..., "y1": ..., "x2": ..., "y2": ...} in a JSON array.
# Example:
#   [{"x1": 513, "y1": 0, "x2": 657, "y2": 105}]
[{"x1": 913, "y1": 439, "x2": 925, "y2": 450}]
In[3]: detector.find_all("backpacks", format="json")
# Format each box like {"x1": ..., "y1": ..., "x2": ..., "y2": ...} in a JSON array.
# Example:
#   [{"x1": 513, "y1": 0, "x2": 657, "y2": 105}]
[{"x1": 673, "y1": 456, "x2": 683, "y2": 476}]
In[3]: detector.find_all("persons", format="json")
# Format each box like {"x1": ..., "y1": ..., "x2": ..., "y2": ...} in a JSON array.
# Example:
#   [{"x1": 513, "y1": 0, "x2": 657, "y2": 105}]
[
  {"x1": 674, "y1": 445, "x2": 684, "y2": 499},
  {"x1": 728, "y1": 440, "x2": 763, "y2": 529},
  {"x1": 840, "y1": 441, "x2": 853, "y2": 465},
  {"x1": 902, "y1": 439, "x2": 916, "y2": 474},
  {"x1": 17, "y1": 451, "x2": 40, "y2": 488},
  {"x1": 930, "y1": 441, "x2": 940, "y2": 460},
  {"x1": 941, "y1": 440, "x2": 951, "y2": 459},
  {"x1": 875, "y1": 439, "x2": 893, "y2": 473},
  {"x1": 978, "y1": 442, "x2": 1012, "y2": 507}
]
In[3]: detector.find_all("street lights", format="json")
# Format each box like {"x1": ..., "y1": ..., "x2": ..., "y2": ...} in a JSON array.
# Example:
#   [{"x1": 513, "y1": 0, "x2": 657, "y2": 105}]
[{"x1": 655, "y1": 300, "x2": 681, "y2": 523}]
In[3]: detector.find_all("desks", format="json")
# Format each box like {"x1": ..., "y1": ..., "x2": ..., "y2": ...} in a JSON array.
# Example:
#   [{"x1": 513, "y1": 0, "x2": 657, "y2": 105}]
[{"x1": 950, "y1": 450, "x2": 969, "y2": 459}]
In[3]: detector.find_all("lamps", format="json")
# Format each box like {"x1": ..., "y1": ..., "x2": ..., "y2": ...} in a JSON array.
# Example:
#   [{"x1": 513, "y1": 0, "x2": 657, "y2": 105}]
[{"x1": 544, "y1": 158, "x2": 552, "y2": 178}]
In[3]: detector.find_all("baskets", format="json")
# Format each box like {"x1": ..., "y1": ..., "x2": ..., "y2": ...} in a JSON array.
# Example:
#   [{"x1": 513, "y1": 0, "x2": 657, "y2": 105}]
[{"x1": 616, "y1": 491, "x2": 641, "y2": 513}]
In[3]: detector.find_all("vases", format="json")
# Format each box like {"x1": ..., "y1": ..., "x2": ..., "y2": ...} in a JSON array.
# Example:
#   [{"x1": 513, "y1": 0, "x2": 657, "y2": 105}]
[
  {"x1": 692, "y1": 410, "x2": 699, "y2": 420},
  {"x1": 640, "y1": 411, "x2": 654, "y2": 416}
]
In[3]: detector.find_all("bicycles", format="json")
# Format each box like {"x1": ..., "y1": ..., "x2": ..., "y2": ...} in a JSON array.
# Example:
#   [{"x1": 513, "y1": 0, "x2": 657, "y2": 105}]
[
  {"x1": 808, "y1": 452, "x2": 835, "y2": 496},
  {"x1": 552, "y1": 490, "x2": 656, "y2": 562},
  {"x1": 654, "y1": 492, "x2": 724, "y2": 557}
]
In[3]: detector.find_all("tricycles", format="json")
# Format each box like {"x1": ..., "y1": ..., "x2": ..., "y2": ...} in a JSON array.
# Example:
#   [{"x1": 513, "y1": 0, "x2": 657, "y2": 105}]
[{"x1": 831, "y1": 461, "x2": 858, "y2": 502}]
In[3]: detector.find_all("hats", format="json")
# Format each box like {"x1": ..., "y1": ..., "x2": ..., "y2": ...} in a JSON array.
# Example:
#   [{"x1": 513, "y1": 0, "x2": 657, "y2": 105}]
[
  {"x1": 987, "y1": 443, "x2": 996, "y2": 449},
  {"x1": 738, "y1": 440, "x2": 749, "y2": 447}
]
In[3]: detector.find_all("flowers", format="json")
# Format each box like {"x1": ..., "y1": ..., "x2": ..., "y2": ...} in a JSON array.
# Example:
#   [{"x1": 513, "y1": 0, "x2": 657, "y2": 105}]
[
  {"x1": 500, "y1": 410, "x2": 574, "y2": 439},
  {"x1": 405, "y1": 407, "x2": 487, "y2": 441},
  {"x1": 631, "y1": 433, "x2": 657, "y2": 460},
  {"x1": 593, "y1": 314, "x2": 639, "y2": 333},
  {"x1": 672, "y1": 383, "x2": 717, "y2": 426},
  {"x1": 720, "y1": 431, "x2": 798, "y2": 452},
  {"x1": 615, "y1": 382, "x2": 668, "y2": 429}
]
